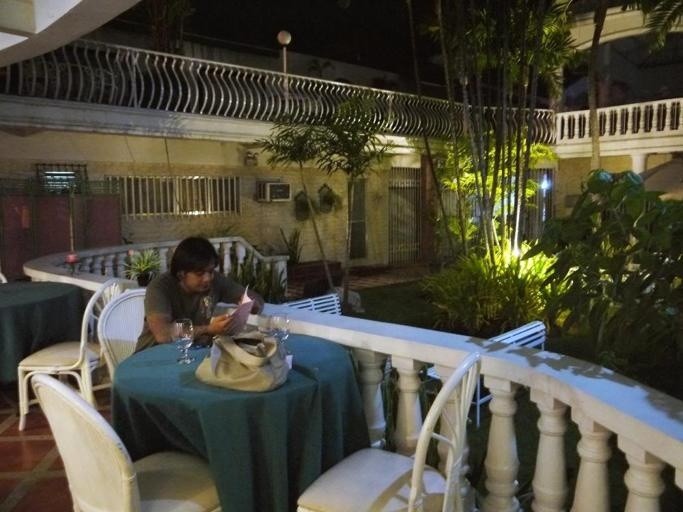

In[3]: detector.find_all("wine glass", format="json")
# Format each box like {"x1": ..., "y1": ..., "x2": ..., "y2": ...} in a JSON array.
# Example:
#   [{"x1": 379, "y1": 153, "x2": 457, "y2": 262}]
[
  {"x1": 257, "y1": 312, "x2": 273, "y2": 337},
  {"x1": 171, "y1": 318, "x2": 196, "y2": 364},
  {"x1": 270, "y1": 313, "x2": 288, "y2": 355}
]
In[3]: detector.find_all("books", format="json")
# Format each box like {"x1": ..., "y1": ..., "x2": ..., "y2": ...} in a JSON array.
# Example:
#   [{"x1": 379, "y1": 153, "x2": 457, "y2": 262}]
[{"x1": 225, "y1": 283, "x2": 255, "y2": 336}]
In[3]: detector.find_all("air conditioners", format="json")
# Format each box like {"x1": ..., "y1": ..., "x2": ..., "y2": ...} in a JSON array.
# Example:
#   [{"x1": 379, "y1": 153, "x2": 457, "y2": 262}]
[{"x1": 258, "y1": 182, "x2": 290, "y2": 203}]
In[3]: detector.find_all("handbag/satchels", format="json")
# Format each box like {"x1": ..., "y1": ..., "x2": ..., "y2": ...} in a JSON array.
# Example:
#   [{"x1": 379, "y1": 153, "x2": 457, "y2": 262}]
[{"x1": 193, "y1": 329, "x2": 295, "y2": 394}]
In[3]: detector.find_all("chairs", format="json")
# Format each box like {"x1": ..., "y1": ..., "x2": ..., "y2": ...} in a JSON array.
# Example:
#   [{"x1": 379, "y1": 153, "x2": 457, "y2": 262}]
[
  {"x1": 297, "y1": 350, "x2": 482, "y2": 512},
  {"x1": 97, "y1": 289, "x2": 147, "y2": 383},
  {"x1": 31, "y1": 375, "x2": 222, "y2": 512},
  {"x1": 17, "y1": 278, "x2": 123, "y2": 432}
]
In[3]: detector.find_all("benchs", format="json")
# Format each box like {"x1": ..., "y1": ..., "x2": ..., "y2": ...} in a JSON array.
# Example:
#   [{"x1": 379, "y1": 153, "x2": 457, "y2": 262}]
[
  {"x1": 282, "y1": 294, "x2": 342, "y2": 316},
  {"x1": 427, "y1": 321, "x2": 547, "y2": 429}
]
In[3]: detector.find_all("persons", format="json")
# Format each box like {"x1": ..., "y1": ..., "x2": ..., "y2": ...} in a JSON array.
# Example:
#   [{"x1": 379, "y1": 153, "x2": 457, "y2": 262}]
[{"x1": 132, "y1": 236, "x2": 262, "y2": 352}]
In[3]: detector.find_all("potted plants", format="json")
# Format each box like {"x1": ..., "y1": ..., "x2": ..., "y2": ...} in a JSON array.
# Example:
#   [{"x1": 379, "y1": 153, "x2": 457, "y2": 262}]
[{"x1": 121, "y1": 248, "x2": 162, "y2": 286}]
